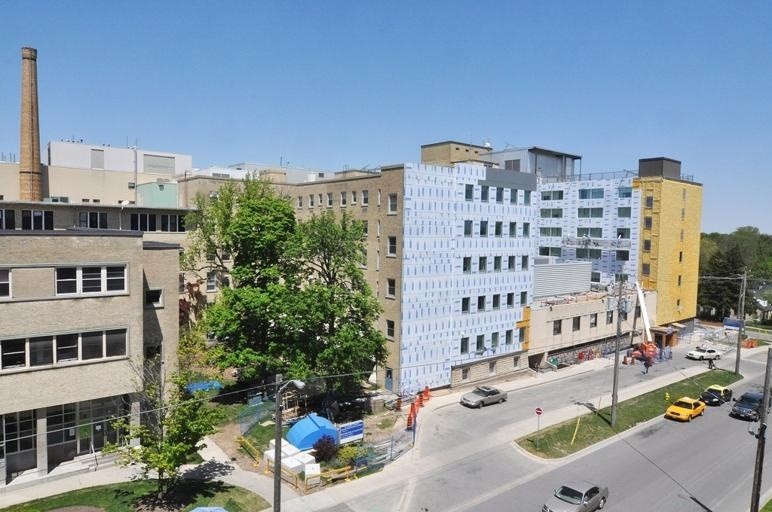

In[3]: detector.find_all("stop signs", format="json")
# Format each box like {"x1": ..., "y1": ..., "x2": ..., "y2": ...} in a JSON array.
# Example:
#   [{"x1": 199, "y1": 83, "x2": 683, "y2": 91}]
[{"x1": 535, "y1": 407, "x2": 543, "y2": 416}]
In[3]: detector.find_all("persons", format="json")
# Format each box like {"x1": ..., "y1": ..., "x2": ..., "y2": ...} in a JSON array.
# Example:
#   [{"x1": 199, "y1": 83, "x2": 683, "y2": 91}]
[
  {"x1": 644, "y1": 356, "x2": 651, "y2": 373},
  {"x1": 708, "y1": 357, "x2": 713, "y2": 369}
]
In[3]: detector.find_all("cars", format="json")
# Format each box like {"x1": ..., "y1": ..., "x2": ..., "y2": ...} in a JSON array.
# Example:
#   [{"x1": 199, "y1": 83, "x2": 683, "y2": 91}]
[
  {"x1": 729, "y1": 391, "x2": 772, "y2": 422},
  {"x1": 665, "y1": 395, "x2": 707, "y2": 423},
  {"x1": 699, "y1": 384, "x2": 733, "y2": 407},
  {"x1": 461, "y1": 384, "x2": 508, "y2": 409},
  {"x1": 686, "y1": 346, "x2": 723, "y2": 362},
  {"x1": 542, "y1": 479, "x2": 609, "y2": 512}
]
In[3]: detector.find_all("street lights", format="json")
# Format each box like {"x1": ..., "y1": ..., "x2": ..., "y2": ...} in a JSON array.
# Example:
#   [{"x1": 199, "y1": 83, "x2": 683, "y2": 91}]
[{"x1": 273, "y1": 371, "x2": 307, "y2": 512}]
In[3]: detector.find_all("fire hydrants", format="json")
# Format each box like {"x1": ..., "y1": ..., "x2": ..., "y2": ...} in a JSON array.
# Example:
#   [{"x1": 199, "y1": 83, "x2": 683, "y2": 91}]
[{"x1": 664, "y1": 392, "x2": 671, "y2": 402}]
